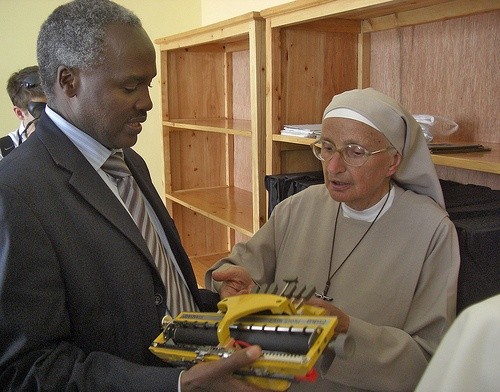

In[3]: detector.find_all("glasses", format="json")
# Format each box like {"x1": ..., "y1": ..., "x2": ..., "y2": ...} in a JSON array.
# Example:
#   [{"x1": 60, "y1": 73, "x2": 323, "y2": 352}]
[
  {"x1": 12, "y1": 73, "x2": 41, "y2": 103},
  {"x1": 309, "y1": 139, "x2": 396, "y2": 167}
]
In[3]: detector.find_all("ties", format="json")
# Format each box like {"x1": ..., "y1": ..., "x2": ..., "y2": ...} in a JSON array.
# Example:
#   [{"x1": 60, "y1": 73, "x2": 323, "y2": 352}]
[{"x1": 100, "y1": 148, "x2": 201, "y2": 318}]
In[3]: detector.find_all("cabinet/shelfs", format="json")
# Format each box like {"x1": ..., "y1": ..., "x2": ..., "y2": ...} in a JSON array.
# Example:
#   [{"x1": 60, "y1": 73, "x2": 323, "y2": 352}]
[
  {"x1": 261, "y1": 0, "x2": 500, "y2": 223},
  {"x1": 154, "y1": 12, "x2": 265, "y2": 289}
]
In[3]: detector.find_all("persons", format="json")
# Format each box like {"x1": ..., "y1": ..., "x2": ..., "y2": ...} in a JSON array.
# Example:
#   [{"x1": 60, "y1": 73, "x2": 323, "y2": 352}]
[
  {"x1": 0, "y1": 66, "x2": 48, "y2": 161},
  {"x1": 0, "y1": 0, "x2": 264, "y2": 392},
  {"x1": 414, "y1": 293, "x2": 500, "y2": 391},
  {"x1": 205, "y1": 86, "x2": 460, "y2": 391}
]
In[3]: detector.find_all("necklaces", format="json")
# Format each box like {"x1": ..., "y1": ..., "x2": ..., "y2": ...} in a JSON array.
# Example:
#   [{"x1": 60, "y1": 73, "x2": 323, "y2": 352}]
[{"x1": 313, "y1": 182, "x2": 390, "y2": 302}]
